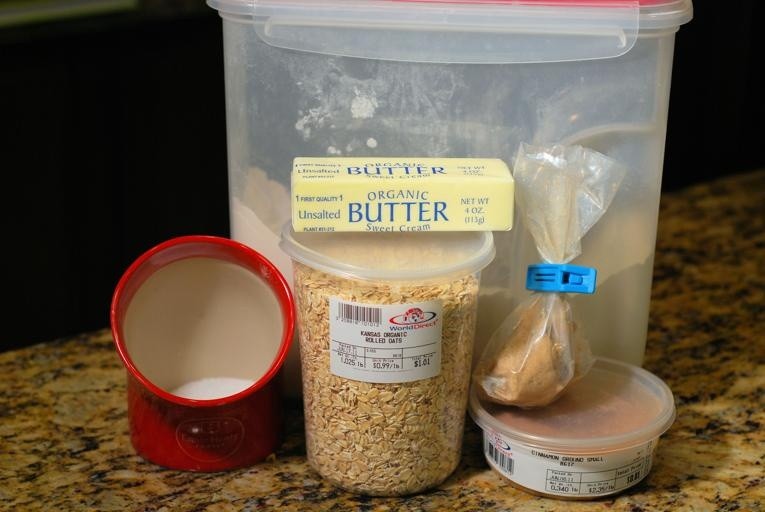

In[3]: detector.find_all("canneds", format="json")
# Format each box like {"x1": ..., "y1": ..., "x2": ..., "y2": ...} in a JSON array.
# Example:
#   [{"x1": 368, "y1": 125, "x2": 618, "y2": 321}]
[{"x1": 280, "y1": 217, "x2": 497, "y2": 498}]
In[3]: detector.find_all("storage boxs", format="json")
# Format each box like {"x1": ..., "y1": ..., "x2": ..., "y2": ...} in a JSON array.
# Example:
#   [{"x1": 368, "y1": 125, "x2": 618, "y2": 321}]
[{"x1": 205, "y1": 2, "x2": 694, "y2": 398}]
